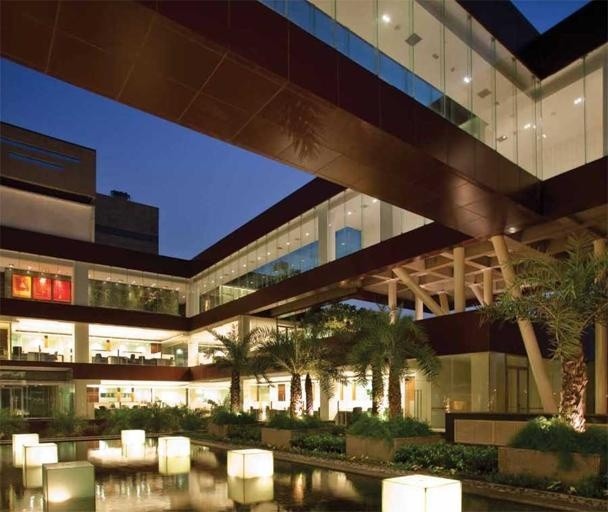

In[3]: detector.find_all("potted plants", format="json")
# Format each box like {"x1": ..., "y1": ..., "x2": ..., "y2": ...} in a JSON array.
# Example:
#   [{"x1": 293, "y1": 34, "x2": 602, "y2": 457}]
[
  {"x1": 475, "y1": 231, "x2": 608, "y2": 491},
  {"x1": 205, "y1": 305, "x2": 444, "y2": 463}
]
